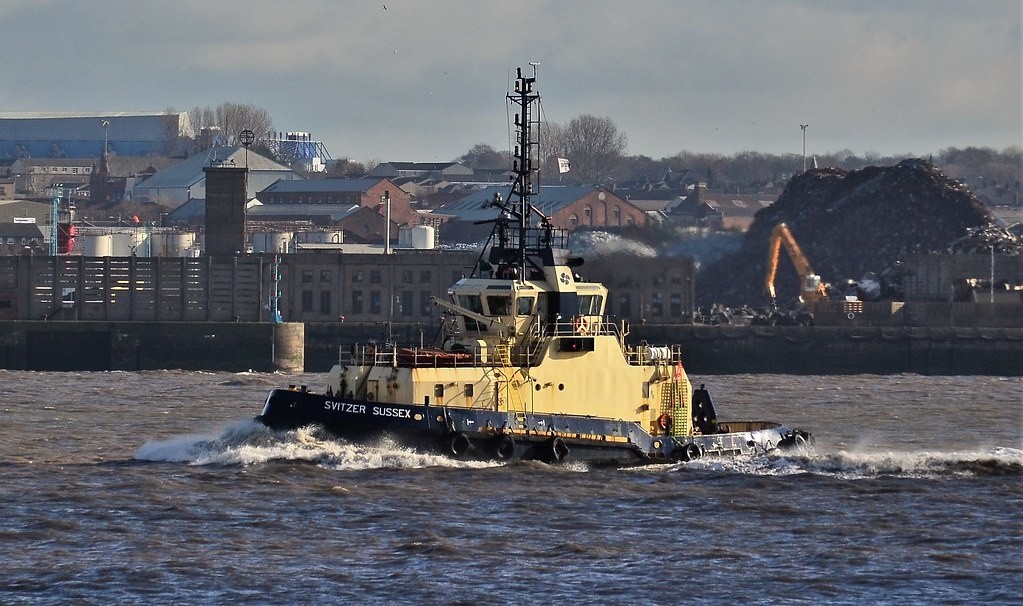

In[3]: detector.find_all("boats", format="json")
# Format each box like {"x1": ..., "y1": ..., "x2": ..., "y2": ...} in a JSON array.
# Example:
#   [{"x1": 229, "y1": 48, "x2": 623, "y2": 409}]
[{"x1": 255, "y1": 59, "x2": 815, "y2": 467}]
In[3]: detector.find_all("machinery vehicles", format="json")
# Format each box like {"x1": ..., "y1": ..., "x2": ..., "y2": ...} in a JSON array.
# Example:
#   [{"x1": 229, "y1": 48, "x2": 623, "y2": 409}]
[{"x1": 760, "y1": 222, "x2": 867, "y2": 325}]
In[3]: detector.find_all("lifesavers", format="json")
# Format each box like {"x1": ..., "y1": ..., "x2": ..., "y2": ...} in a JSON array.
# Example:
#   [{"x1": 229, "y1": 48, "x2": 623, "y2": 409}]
[
  {"x1": 660, "y1": 413, "x2": 673, "y2": 430},
  {"x1": 575, "y1": 318, "x2": 588, "y2": 332}
]
